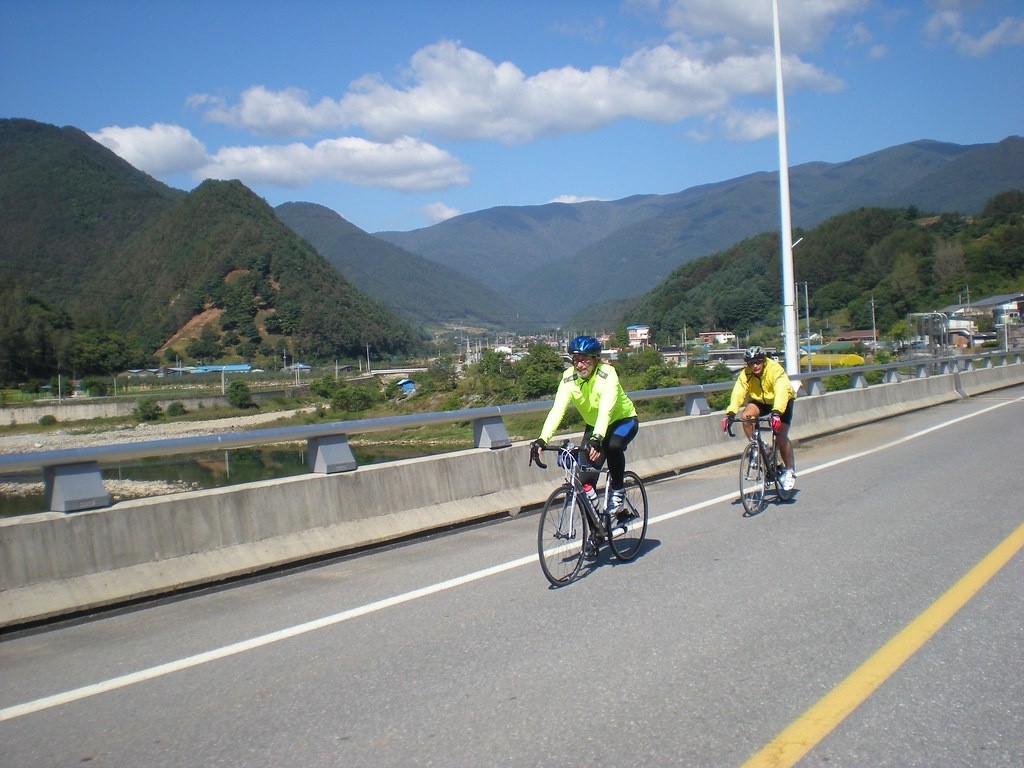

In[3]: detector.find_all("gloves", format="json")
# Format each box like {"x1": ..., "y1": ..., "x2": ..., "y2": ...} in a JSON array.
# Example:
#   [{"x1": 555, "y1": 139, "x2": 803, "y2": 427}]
[
  {"x1": 770, "y1": 414, "x2": 782, "y2": 433},
  {"x1": 722, "y1": 415, "x2": 729, "y2": 431}
]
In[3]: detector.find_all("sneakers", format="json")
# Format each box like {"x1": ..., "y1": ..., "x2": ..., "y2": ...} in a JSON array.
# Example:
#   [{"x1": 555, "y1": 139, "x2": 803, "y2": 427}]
[
  {"x1": 607, "y1": 487, "x2": 626, "y2": 515},
  {"x1": 750, "y1": 442, "x2": 769, "y2": 469},
  {"x1": 784, "y1": 467, "x2": 795, "y2": 492},
  {"x1": 581, "y1": 532, "x2": 605, "y2": 558}
]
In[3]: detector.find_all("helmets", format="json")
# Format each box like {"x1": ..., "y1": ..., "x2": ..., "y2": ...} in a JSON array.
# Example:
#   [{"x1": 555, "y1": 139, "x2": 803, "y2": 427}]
[
  {"x1": 567, "y1": 335, "x2": 601, "y2": 357},
  {"x1": 744, "y1": 345, "x2": 766, "y2": 362}
]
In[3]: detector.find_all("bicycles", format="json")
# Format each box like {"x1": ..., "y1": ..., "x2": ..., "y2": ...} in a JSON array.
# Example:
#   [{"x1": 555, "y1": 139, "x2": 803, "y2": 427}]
[
  {"x1": 529, "y1": 438, "x2": 649, "y2": 586},
  {"x1": 726, "y1": 410, "x2": 795, "y2": 515}
]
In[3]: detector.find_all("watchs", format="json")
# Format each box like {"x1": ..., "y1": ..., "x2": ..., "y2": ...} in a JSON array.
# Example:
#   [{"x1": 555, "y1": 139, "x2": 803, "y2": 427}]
[{"x1": 591, "y1": 433, "x2": 603, "y2": 442}]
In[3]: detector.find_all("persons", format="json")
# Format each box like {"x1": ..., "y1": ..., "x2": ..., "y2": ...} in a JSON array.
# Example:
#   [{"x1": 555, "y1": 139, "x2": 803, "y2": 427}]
[
  {"x1": 529, "y1": 335, "x2": 639, "y2": 560},
  {"x1": 722, "y1": 346, "x2": 798, "y2": 491}
]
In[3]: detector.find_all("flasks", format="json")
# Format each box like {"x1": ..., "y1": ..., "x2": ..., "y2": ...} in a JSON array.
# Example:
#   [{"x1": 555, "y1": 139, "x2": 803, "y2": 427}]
[{"x1": 584, "y1": 484, "x2": 601, "y2": 511}]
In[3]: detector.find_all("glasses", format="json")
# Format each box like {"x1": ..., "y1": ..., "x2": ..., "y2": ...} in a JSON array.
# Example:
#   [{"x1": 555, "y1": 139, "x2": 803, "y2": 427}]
[
  {"x1": 572, "y1": 356, "x2": 597, "y2": 364},
  {"x1": 748, "y1": 357, "x2": 765, "y2": 366}
]
